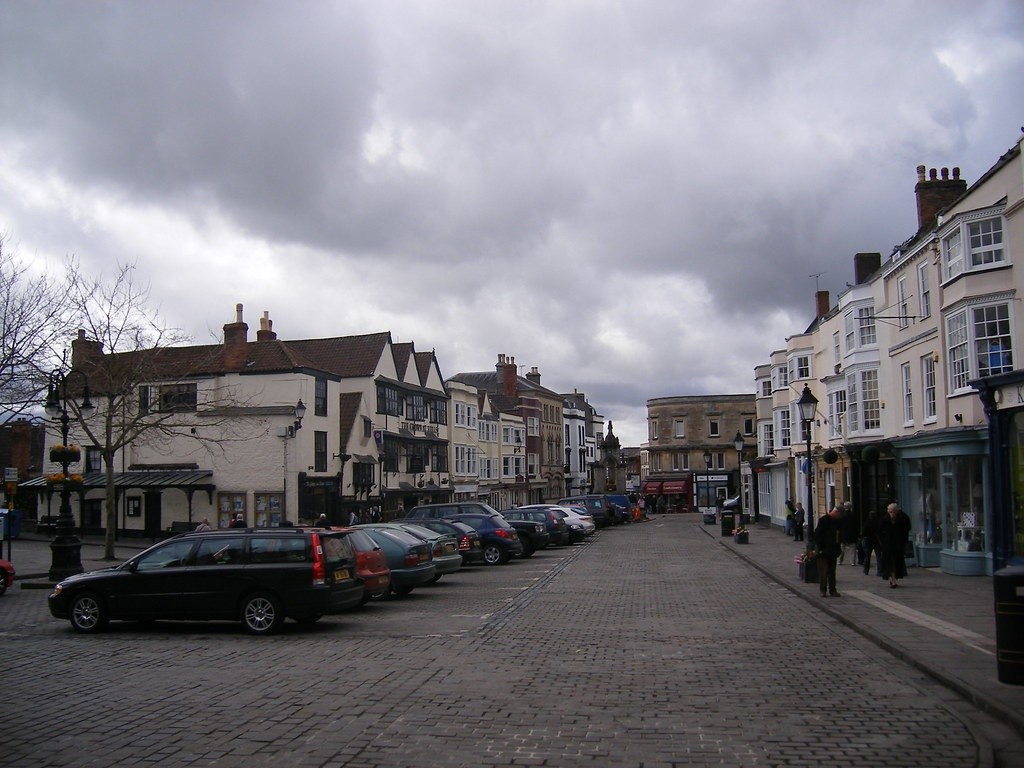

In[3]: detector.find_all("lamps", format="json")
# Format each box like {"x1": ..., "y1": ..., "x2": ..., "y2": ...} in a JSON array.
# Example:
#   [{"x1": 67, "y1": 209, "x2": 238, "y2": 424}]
[
  {"x1": 513, "y1": 437, "x2": 522, "y2": 454},
  {"x1": 294, "y1": 399, "x2": 306, "y2": 429}
]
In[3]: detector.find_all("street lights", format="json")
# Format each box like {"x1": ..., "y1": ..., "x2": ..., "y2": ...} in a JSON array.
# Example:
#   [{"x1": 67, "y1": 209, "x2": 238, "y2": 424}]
[
  {"x1": 733, "y1": 429, "x2": 749, "y2": 544},
  {"x1": 796, "y1": 382, "x2": 823, "y2": 584},
  {"x1": 703, "y1": 448, "x2": 716, "y2": 525},
  {"x1": 42, "y1": 369, "x2": 96, "y2": 581}
]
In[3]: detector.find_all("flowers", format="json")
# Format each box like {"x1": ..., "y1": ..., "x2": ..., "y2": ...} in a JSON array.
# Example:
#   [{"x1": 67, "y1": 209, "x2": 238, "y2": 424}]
[
  {"x1": 793, "y1": 549, "x2": 818, "y2": 564},
  {"x1": 45, "y1": 473, "x2": 82, "y2": 483},
  {"x1": 732, "y1": 527, "x2": 749, "y2": 535},
  {"x1": 48, "y1": 444, "x2": 79, "y2": 452}
]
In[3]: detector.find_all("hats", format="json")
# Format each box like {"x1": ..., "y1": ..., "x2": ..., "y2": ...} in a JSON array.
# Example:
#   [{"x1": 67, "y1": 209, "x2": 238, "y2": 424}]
[{"x1": 836, "y1": 505, "x2": 845, "y2": 517}]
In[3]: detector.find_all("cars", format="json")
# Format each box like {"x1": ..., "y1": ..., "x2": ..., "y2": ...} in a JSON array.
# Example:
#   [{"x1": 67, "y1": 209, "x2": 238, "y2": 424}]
[{"x1": 300, "y1": 494, "x2": 641, "y2": 606}]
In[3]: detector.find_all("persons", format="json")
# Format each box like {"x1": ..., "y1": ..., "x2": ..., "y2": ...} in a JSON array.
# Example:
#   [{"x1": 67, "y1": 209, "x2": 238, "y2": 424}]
[
  {"x1": 316, "y1": 513, "x2": 333, "y2": 526},
  {"x1": 861, "y1": 501, "x2": 881, "y2": 576},
  {"x1": 815, "y1": 504, "x2": 843, "y2": 598},
  {"x1": 195, "y1": 517, "x2": 211, "y2": 531},
  {"x1": 347, "y1": 507, "x2": 381, "y2": 527},
  {"x1": 715, "y1": 495, "x2": 724, "y2": 519},
  {"x1": 229, "y1": 514, "x2": 247, "y2": 528},
  {"x1": 880, "y1": 503, "x2": 911, "y2": 590},
  {"x1": 784, "y1": 498, "x2": 795, "y2": 535},
  {"x1": 837, "y1": 501, "x2": 858, "y2": 566},
  {"x1": 793, "y1": 502, "x2": 805, "y2": 541}
]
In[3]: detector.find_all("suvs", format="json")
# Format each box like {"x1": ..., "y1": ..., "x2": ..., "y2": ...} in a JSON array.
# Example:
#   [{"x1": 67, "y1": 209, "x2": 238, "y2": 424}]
[{"x1": 48, "y1": 528, "x2": 365, "y2": 636}]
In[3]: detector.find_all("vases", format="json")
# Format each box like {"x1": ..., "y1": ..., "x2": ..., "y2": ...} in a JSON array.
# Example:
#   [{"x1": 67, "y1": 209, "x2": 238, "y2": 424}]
[
  {"x1": 50, "y1": 451, "x2": 80, "y2": 462},
  {"x1": 797, "y1": 562, "x2": 818, "y2": 583},
  {"x1": 733, "y1": 534, "x2": 748, "y2": 544}
]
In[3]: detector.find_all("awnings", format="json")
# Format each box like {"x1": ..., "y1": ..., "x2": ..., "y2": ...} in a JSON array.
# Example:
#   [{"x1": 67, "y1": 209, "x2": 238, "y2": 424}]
[
  {"x1": 640, "y1": 482, "x2": 688, "y2": 495},
  {"x1": 15, "y1": 469, "x2": 211, "y2": 488}
]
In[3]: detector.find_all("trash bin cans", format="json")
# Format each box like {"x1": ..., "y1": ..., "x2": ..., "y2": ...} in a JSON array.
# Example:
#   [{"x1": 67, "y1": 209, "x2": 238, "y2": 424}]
[
  {"x1": 721, "y1": 510, "x2": 736, "y2": 536},
  {"x1": 0, "y1": 509, "x2": 23, "y2": 538},
  {"x1": 993, "y1": 566, "x2": 1024, "y2": 686}
]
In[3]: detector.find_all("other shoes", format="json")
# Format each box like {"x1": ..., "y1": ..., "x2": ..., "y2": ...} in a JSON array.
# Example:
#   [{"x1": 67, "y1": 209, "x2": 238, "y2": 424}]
[
  {"x1": 838, "y1": 560, "x2": 841, "y2": 565},
  {"x1": 820, "y1": 591, "x2": 826, "y2": 597},
  {"x1": 829, "y1": 590, "x2": 840, "y2": 597},
  {"x1": 890, "y1": 580, "x2": 897, "y2": 588},
  {"x1": 852, "y1": 563, "x2": 854, "y2": 566}
]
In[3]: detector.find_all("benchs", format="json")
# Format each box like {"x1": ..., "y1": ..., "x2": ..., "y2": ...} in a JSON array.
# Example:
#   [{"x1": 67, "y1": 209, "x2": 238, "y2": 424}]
[
  {"x1": 164, "y1": 521, "x2": 201, "y2": 540},
  {"x1": 34, "y1": 515, "x2": 59, "y2": 535}
]
